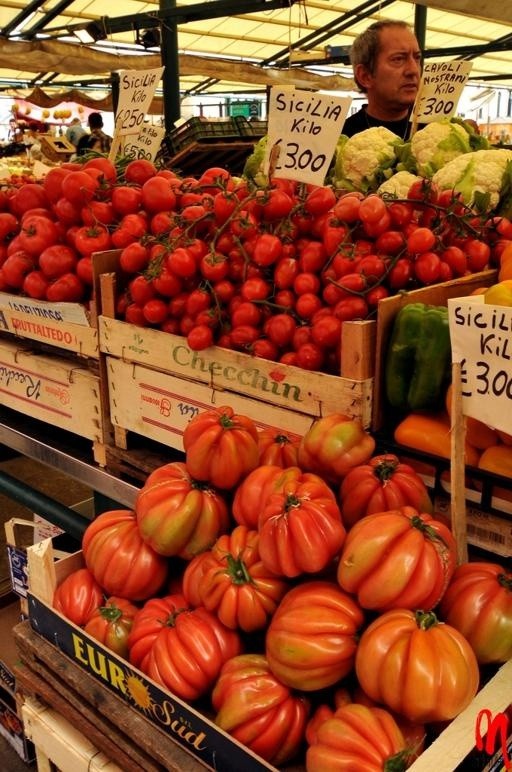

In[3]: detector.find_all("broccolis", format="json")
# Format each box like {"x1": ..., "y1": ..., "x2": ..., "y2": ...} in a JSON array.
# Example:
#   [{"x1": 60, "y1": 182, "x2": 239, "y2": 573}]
[{"x1": 245, "y1": 119, "x2": 512, "y2": 216}]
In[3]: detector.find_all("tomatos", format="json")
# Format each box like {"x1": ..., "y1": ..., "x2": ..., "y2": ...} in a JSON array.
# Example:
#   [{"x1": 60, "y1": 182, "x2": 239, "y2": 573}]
[
  {"x1": 52, "y1": 405, "x2": 512, "y2": 772},
  {"x1": 0, "y1": 156, "x2": 512, "y2": 371}
]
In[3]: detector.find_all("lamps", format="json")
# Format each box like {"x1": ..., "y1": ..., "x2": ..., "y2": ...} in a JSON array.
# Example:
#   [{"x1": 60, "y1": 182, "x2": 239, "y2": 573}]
[
  {"x1": 136, "y1": 27, "x2": 161, "y2": 48},
  {"x1": 71, "y1": 21, "x2": 106, "y2": 44}
]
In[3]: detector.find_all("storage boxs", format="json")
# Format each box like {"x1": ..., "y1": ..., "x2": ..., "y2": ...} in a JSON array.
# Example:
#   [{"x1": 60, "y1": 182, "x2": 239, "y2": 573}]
[{"x1": 41, "y1": 135, "x2": 78, "y2": 162}]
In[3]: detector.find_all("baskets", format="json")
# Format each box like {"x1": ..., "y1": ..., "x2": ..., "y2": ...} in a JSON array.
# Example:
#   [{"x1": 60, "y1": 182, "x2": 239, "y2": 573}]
[{"x1": 160, "y1": 113, "x2": 268, "y2": 163}]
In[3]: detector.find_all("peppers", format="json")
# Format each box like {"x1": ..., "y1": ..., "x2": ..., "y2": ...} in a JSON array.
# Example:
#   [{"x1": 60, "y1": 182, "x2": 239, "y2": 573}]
[{"x1": 384, "y1": 245, "x2": 512, "y2": 481}]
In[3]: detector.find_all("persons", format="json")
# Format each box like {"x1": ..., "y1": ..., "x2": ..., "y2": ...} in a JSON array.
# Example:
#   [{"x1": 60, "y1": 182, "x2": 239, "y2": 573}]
[
  {"x1": 342, "y1": 20, "x2": 425, "y2": 139},
  {"x1": 79, "y1": 112, "x2": 112, "y2": 154},
  {"x1": 77, "y1": 134, "x2": 102, "y2": 158},
  {"x1": 66, "y1": 118, "x2": 86, "y2": 145},
  {"x1": 13, "y1": 120, "x2": 27, "y2": 141},
  {"x1": 7, "y1": 118, "x2": 16, "y2": 141}
]
